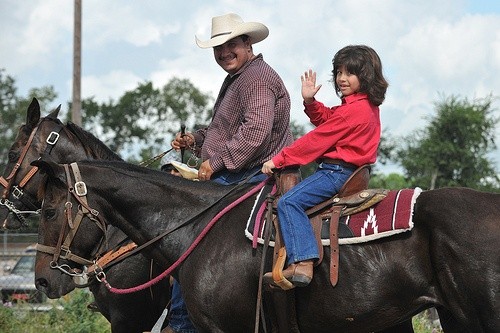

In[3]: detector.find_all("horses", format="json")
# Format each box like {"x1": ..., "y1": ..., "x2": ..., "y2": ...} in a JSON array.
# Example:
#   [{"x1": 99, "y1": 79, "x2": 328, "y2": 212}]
[
  {"x1": 28, "y1": 149, "x2": 500, "y2": 333},
  {"x1": 0, "y1": 96, "x2": 175, "y2": 333}
]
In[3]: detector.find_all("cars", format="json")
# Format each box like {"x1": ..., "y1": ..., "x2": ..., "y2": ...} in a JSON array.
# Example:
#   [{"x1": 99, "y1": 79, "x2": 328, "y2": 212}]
[{"x1": 0, "y1": 246, "x2": 48, "y2": 305}]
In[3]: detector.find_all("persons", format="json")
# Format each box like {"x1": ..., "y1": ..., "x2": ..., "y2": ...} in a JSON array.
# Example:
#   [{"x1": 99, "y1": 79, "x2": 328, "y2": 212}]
[
  {"x1": 161, "y1": 162, "x2": 184, "y2": 178},
  {"x1": 160, "y1": 13, "x2": 304, "y2": 333},
  {"x1": 262, "y1": 43, "x2": 391, "y2": 291}
]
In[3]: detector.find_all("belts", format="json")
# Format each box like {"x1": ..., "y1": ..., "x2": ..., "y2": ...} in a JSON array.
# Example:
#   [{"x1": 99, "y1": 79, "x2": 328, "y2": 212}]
[{"x1": 320, "y1": 158, "x2": 356, "y2": 169}]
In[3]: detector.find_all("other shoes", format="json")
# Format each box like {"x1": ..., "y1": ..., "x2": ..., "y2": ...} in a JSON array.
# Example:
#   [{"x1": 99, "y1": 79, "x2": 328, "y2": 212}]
[
  {"x1": 142, "y1": 324, "x2": 175, "y2": 333},
  {"x1": 264, "y1": 260, "x2": 313, "y2": 288}
]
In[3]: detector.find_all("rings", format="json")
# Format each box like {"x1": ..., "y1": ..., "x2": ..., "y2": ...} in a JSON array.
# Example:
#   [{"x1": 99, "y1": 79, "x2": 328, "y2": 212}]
[{"x1": 202, "y1": 172, "x2": 206, "y2": 176}]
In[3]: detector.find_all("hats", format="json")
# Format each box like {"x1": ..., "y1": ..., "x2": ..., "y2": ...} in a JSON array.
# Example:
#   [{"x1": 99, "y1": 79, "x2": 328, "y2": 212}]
[{"x1": 195, "y1": 14, "x2": 269, "y2": 49}]
